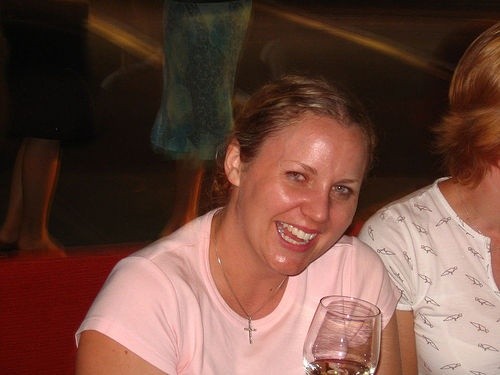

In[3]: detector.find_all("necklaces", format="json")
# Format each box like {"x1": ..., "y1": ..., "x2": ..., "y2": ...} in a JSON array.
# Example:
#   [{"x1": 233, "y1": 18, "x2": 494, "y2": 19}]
[{"x1": 213, "y1": 206, "x2": 289, "y2": 345}]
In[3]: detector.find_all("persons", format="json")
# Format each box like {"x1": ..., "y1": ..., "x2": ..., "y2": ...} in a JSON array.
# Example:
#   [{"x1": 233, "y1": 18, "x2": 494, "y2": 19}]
[
  {"x1": 149, "y1": 0, "x2": 254, "y2": 241},
  {"x1": 357, "y1": 19, "x2": 500, "y2": 375},
  {"x1": 74, "y1": 69, "x2": 403, "y2": 375},
  {"x1": 0, "y1": 0, "x2": 100, "y2": 259}
]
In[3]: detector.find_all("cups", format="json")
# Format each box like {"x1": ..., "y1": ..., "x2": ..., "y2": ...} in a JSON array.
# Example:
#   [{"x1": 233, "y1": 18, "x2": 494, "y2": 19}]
[{"x1": 302, "y1": 295, "x2": 382, "y2": 375}]
